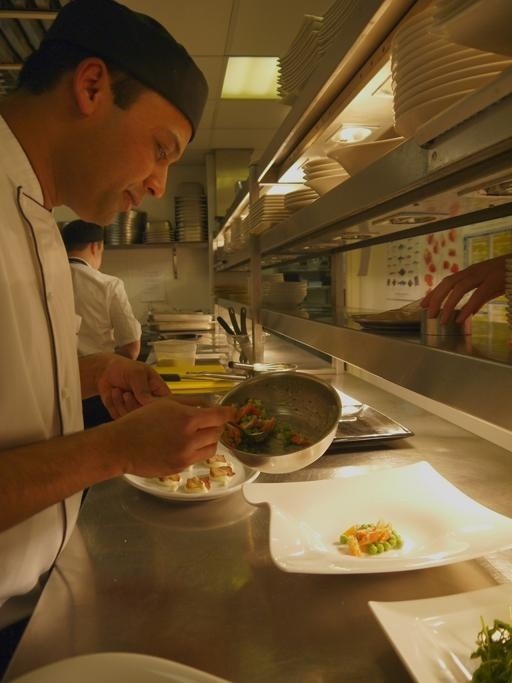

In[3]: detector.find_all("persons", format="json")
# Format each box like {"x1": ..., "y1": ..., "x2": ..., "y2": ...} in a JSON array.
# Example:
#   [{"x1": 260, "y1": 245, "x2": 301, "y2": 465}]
[
  {"x1": 421, "y1": 252, "x2": 511, "y2": 324},
  {"x1": 0, "y1": 0, "x2": 237, "y2": 683},
  {"x1": 61, "y1": 218, "x2": 142, "y2": 430}
]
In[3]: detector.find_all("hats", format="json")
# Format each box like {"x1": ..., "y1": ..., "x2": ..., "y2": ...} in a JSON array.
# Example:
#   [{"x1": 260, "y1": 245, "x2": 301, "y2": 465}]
[
  {"x1": 39, "y1": 0, "x2": 209, "y2": 143},
  {"x1": 62, "y1": 220, "x2": 105, "y2": 242}
]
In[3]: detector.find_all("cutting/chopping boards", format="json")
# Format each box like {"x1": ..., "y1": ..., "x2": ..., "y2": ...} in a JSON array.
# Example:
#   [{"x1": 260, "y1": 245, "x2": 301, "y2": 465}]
[{"x1": 153, "y1": 365, "x2": 232, "y2": 395}]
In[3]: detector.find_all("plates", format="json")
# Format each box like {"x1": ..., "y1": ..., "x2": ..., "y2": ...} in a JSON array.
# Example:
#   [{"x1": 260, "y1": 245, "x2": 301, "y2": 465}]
[
  {"x1": 353, "y1": 317, "x2": 422, "y2": 333},
  {"x1": 222, "y1": 188, "x2": 322, "y2": 258},
  {"x1": 362, "y1": 581, "x2": 512, "y2": 683},
  {"x1": 242, "y1": 459, "x2": 512, "y2": 577},
  {"x1": 121, "y1": 438, "x2": 262, "y2": 506},
  {"x1": 273, "y1": 0, "x2": 366, "y2": 108},
  {"x1": 390, "y1": 0, "x2": 512, "y2": 135}
]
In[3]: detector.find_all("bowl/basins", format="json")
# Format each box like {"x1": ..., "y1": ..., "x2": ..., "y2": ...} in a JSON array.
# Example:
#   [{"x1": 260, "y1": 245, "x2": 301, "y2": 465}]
[
  {"x1": 326, "y1": 134, "x2": 407, "y2": 177},
  {"x1": 301, "y1": 154, "x2": 351, "y2": 195},
  {"x1": 272, "y1": 280, "x2": 308, "y2": 305},
  {"x1": 211, "y1": 369, "x2": 342, "y2": 476}
]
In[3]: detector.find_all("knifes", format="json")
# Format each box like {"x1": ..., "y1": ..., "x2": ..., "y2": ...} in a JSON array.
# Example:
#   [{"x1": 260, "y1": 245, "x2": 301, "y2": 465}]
[
  {"x1": 240, "y1": 307, "x2": 253, "y2": 361},
  {"x1": 158, "y1": 373, "x2": 225, "y2": 384}
]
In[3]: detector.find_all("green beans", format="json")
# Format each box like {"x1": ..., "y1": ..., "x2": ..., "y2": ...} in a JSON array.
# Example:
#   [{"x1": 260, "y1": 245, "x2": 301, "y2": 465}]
[{"x1": 339, "y1": 523, "x2": 403, "y2": 552}]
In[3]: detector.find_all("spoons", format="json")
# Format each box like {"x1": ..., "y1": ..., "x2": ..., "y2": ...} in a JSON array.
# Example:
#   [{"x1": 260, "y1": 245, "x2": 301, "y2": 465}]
[{"x1": 223, "y1": 418, "x2": 265, "y2": 440}]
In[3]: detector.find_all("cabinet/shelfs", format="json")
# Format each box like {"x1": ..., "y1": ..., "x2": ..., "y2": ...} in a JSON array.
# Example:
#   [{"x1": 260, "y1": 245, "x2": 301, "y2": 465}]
[{"x1": 207, "y1": 3, "x2": 512, "y2": 459}]
